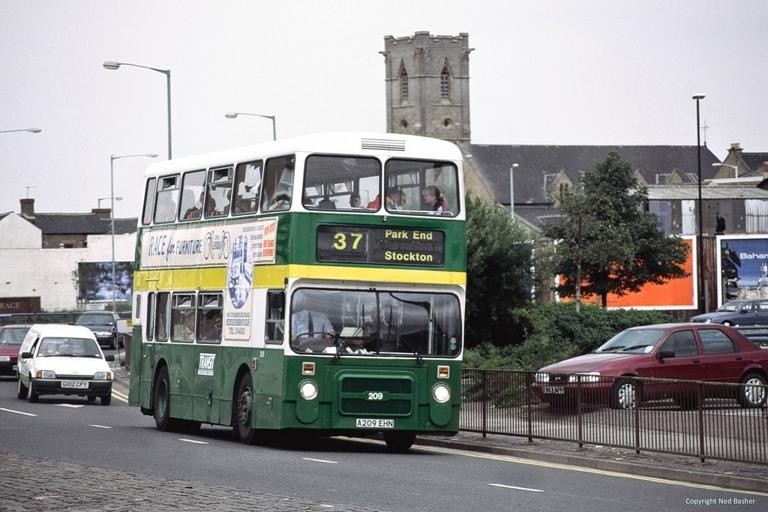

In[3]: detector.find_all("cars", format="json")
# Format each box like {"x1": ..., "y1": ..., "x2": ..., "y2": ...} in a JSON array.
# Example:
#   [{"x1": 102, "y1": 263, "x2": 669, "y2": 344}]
[
  {"x1": 74, "y1": 310, "x2": 126, "y2": 349},
  {"x1": 0, "y1": 324, "x2": 34, "y2": 376},
  {"x1": 17, "y1": 323, "x2": 115, "y2": 406},
  {"x1": 532, "y1": 323, "x2": 767, "y2": 411},
  {"x1": 690, "y1": 298, "x2": 768, "y2": 327}
]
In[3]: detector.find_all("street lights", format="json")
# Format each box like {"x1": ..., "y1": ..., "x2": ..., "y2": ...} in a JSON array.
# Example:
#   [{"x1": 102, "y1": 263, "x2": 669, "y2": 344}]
[
  {"x1": 97, "y1": 196, "x2": 122, "y2": 208},
  {"x1": 223, "y1": 109, "x2": 277, "y2": 142},
  {"x1": 509, "y1": 162, "x2": 519, "y2": 215},
  {"x1": 110, "y1": 153, "x2": 159, "y2": 312},
  {"x1": 0, "y1": 126, "x2": 44, "y2": 134},
  {"x1": 711, "y1": 162, "x2": 738, "y2": 178},
  {"x1": 690, "y1": 91, "x2": 705, "y2": 312},
  {"x1": 103, "y1": 59, "x2": 172, "y2": 161}
]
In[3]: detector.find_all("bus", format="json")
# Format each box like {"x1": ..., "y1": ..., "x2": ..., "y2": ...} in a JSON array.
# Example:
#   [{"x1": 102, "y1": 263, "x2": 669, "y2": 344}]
[{"x1": 127, "y1": 134, "x2": 470, "y2": 449}]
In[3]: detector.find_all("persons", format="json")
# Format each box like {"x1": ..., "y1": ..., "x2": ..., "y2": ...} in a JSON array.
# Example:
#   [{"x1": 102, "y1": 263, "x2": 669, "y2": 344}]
[
  {"x1": 173, "y1": 309, "x2": 223, "y2": 343},
  {"x1": 367, "y1": 186, "x2": 408, "y2": 211},
  {"x1": 422, "y1": 184, "x2": 447, "y2": 215},
  {"x1": 291, "y1": 294, "x2": 335, "y2": 345},
  {"x1": 147, "y1": 188, "x2": 250, "y2": 224},
  {"x1": 349, "y1": 194, "x2": 361, "y2": 208},
  {"x1": 363, "y1": 307, "x2": 388, "y2": 334}
]
